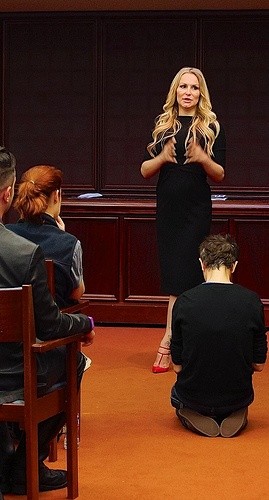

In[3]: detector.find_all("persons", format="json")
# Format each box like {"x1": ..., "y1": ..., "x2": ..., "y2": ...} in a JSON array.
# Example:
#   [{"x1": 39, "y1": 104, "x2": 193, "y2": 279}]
[
  {"x1": 140, "y1": 67, "x2": 226, "y2": 373},
  {"x1": 4, "y1": 165, "x2": 86, "y2": 308},
  {"x1": 169, "y1": 233, "x2": 268, "y2": 438},
  {"x1": 0, "y1": 147, "x2": 96, "y2": 495}
]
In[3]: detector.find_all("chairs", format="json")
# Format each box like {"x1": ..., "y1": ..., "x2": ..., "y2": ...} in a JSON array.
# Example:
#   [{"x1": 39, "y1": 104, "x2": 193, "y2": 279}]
[
  {"x1": 0, "y1": 283, "x2": 87, "y2": 500},
  {"x1": 45, "y1": 258, "x2": 90, "y2": 462}
]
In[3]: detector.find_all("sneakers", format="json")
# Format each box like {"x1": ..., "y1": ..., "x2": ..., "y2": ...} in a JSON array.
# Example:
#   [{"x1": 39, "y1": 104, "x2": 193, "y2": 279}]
[
  {"x1": 0, "y1": 460, "x2": 68, "y2": 495},
  {"x1": 221, "y1": 408, "x2": 248, "y2": 437},
  {"x1": 176, "y1": 406, "x2": 220, "y2": 437}
]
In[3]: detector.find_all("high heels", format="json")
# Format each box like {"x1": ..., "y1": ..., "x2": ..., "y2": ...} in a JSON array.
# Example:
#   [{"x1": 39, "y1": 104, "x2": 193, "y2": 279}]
[{"x1": 152, "y1": 346, "x2": 171, "y2": 373}]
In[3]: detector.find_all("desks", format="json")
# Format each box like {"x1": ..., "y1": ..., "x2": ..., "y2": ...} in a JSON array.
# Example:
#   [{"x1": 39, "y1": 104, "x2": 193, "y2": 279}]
[{"x1": 59, "y1": 197, "x2": 269, "y2": 325}]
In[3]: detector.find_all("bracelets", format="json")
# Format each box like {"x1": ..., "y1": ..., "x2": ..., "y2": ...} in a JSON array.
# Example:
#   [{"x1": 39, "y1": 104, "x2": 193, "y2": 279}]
[{"x1": 88, "y1": 316, "x2": 95, "y2": 330}]
